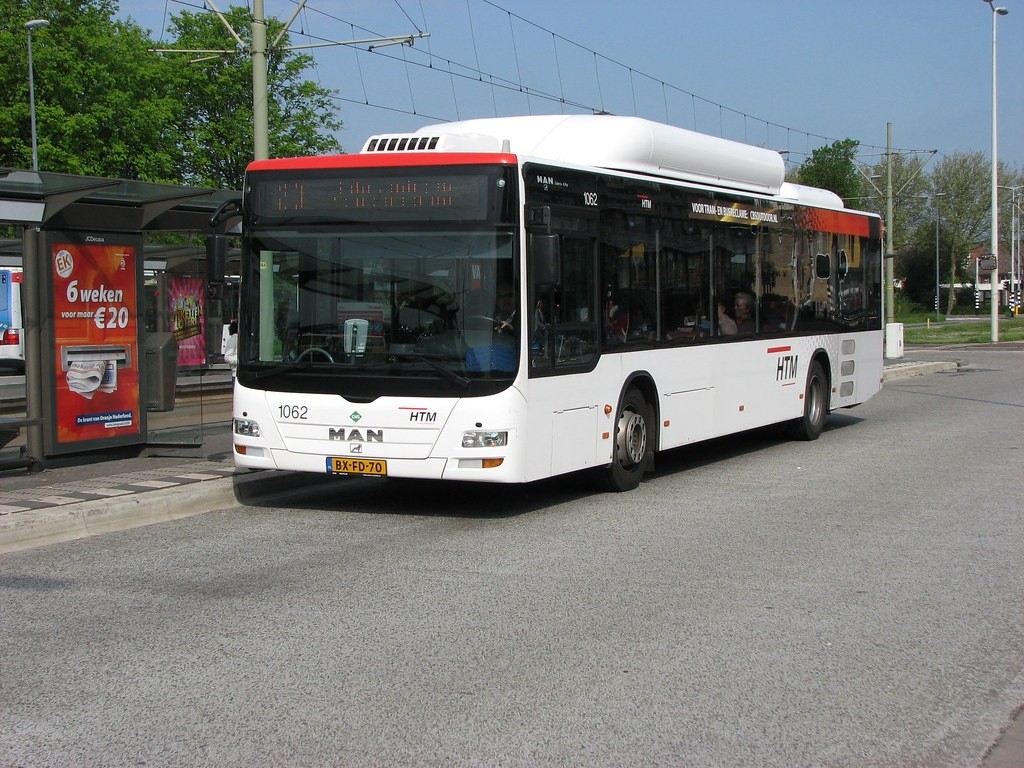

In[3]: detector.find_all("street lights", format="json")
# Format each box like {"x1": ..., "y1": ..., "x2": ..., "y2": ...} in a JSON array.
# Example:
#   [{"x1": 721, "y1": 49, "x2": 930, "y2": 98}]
[
  {"x1": 24, "y1": 18, "x2": 51, "y2": 173},
  {"x1": 936, "y1": 193, "x2": 945, "y2": 323},
  {"x1": 982, "y1": 0, "x2": 1009, "y2": 346},
  {"x1": 997, "y1": 186, "x2": 1024, "y2": 319}
]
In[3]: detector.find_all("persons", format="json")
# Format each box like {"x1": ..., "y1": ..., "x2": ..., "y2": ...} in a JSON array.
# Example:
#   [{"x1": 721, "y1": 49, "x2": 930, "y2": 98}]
[
  {"x1": 224, "y1": 319, "x2": 238, "y2": 389},
  {"x1": 483, "y1": 285, "x2": 515, "y2": 336},
  {"x1": 609, "y1": 291, "x2": 755, "y2": 341}
]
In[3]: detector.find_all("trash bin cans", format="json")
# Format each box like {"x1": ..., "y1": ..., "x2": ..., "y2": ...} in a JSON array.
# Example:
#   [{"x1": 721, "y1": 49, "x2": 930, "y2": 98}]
[{"x1": 144, "y1": 332, "x2": 179, "y2": 412}]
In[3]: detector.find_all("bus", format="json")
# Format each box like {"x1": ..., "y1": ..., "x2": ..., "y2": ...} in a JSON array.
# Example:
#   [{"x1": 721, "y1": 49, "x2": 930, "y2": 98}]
[{"x1": 206, "y1": 116, "x2": 882, "y2": 493}]
